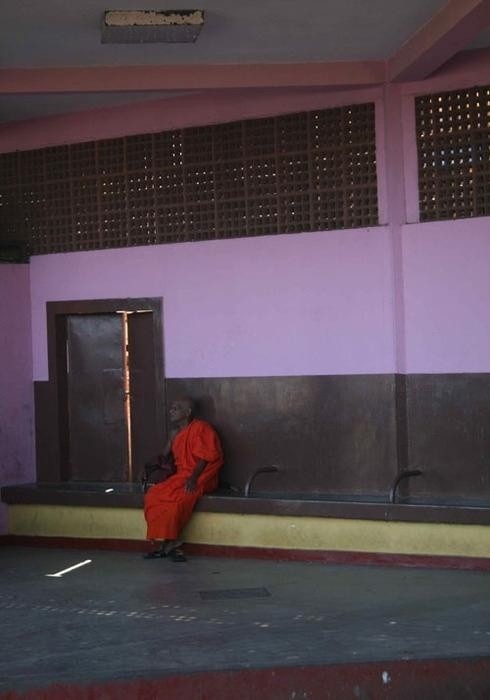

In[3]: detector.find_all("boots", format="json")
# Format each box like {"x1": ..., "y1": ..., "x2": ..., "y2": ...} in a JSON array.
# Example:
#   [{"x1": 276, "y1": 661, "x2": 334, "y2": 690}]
[
  {"x1": 165, "y1": 543, "x2": 185, "y2": 563},
  {"x1": 144, "y1": 541, "x2": 164, "y2": 558}
]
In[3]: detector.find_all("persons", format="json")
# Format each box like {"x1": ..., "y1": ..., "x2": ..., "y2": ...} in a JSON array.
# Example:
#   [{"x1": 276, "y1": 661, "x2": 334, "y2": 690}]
[{"x1": 143, "y1": 400, "x2": 224, "y2": 561}]
